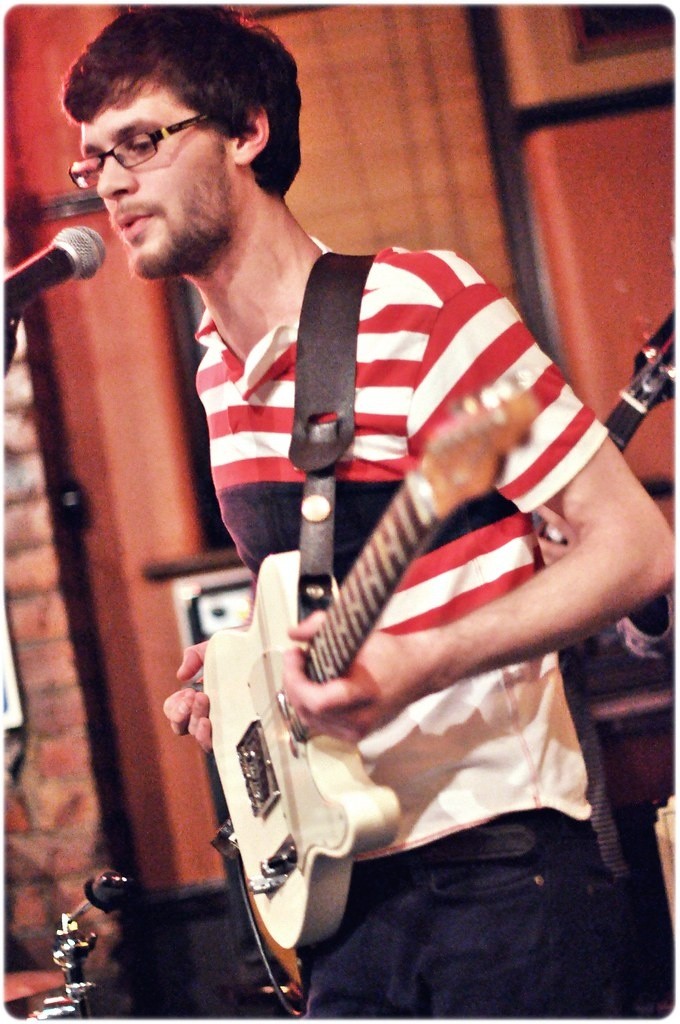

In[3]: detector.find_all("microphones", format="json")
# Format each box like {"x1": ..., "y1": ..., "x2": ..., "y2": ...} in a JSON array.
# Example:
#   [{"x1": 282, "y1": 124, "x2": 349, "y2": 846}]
[{"x1": 4, "y1": 224, "x2": 105, "y2": 321}]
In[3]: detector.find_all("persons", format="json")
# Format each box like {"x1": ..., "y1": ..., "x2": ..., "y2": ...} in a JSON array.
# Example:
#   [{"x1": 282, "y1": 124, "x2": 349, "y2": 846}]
[{"x1": 65, "y1": 6, "x2": 674, "y2": 1019}]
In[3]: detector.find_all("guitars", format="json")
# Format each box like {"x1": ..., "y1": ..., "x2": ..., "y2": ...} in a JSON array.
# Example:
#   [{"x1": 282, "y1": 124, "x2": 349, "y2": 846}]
[
  {"x1": 201, "y1": 371, "x2": 541, "y2": 951},
  {"x1": 604, "y1": 306, "x2": 676, "y2": 451}
]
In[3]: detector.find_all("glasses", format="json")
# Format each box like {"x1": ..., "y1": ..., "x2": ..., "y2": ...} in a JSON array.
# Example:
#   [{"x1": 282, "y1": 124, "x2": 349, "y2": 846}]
[{"x1": 68, "y1": 113, "x2": 210, "y2": 190}]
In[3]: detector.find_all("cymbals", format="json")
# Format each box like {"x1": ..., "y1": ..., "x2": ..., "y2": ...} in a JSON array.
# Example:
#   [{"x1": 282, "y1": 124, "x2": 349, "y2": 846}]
[{"x1": 4, "y1": 964, "x2": 68, "y2": 1006}]
